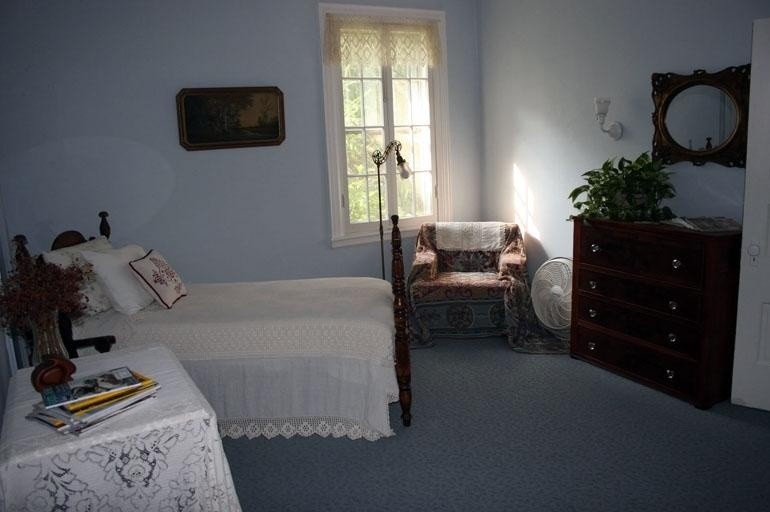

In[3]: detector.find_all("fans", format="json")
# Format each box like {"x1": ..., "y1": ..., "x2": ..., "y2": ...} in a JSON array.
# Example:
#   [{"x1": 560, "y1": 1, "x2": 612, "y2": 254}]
[{"x1": 530, "y1": 254, "x2": 574, "y2": 348}]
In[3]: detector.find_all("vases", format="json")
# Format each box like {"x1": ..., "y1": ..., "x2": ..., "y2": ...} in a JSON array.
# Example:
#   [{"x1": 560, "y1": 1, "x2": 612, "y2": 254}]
[{"x1": 28, "y1": 307, "x2": 70, "y2": 368}]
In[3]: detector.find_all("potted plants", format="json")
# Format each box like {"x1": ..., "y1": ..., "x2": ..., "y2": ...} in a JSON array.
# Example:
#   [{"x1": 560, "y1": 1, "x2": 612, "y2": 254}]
[{"x1": 566, "y1": 151, "x2": 678, "y2": 228}]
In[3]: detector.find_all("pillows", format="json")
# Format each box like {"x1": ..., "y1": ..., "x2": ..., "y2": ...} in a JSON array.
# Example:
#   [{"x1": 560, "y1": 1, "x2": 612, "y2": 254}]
[
  {"x1": 80, "y1": 241, "x2": 155, "y2": 315},
  {"x1": 40, "y1": 234, "x2": 117, "y2": 326},
  {"x1": 129, "y1": 249, "x2": 189, "y2": 311}
]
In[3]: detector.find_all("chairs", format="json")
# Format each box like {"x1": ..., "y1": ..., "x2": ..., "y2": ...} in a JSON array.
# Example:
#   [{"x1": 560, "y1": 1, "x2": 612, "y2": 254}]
[{"x1": 406, "y1": 222, "x2": 529, "y2": 348}]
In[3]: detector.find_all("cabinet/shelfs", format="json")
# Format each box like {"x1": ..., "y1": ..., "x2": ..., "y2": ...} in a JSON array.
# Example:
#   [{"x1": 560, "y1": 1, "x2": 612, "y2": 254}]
[{"x1": 569, "y1": 212, "x2": 741, "y2": 413}]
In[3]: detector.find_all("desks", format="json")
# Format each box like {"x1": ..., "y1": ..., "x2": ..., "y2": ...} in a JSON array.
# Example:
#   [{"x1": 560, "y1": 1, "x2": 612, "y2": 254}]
[{"x1": 1, "y1": 338, "x2": 244, "y2": 511}]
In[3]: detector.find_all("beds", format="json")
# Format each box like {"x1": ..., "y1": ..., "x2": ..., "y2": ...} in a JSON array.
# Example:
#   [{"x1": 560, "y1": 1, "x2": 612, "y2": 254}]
[{"x1": 10, "y1": 210, "x2": 414, "y2": 442}]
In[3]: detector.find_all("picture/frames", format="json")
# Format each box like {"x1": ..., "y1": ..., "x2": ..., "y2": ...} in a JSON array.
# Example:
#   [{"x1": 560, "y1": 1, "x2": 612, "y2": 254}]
[{"x1": 176, "y1": 86, "x2": 287, "y2": 152}]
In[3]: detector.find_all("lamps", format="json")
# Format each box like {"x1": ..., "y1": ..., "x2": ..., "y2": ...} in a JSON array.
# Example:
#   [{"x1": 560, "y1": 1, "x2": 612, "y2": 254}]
[
  {"x1": 372, "y1": 141, "x2": 415, "y2": 280},
  {"x1": 588, "y1": 95, "x2": 625, "y2": 142}
]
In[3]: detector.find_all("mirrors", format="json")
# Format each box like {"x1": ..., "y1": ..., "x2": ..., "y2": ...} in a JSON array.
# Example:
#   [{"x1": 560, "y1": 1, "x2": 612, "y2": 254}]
[{"x1": 651, "y1": 60, "x2": 747, "y2": 173}]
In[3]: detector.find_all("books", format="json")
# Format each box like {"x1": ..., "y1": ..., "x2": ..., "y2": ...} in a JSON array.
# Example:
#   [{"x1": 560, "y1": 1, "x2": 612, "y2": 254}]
[
  {"x1": 672, "y1": 215, "x2": 744, "y2": 232},
  {"x1": 22, "y1": 364, "x2": 163, "y2": 438}
]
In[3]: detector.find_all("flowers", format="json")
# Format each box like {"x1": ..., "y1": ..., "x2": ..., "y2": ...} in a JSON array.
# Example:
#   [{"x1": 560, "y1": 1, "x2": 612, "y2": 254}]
[{"x1": 0, "y1": 251, "x2": 93, "y2": 335}]
